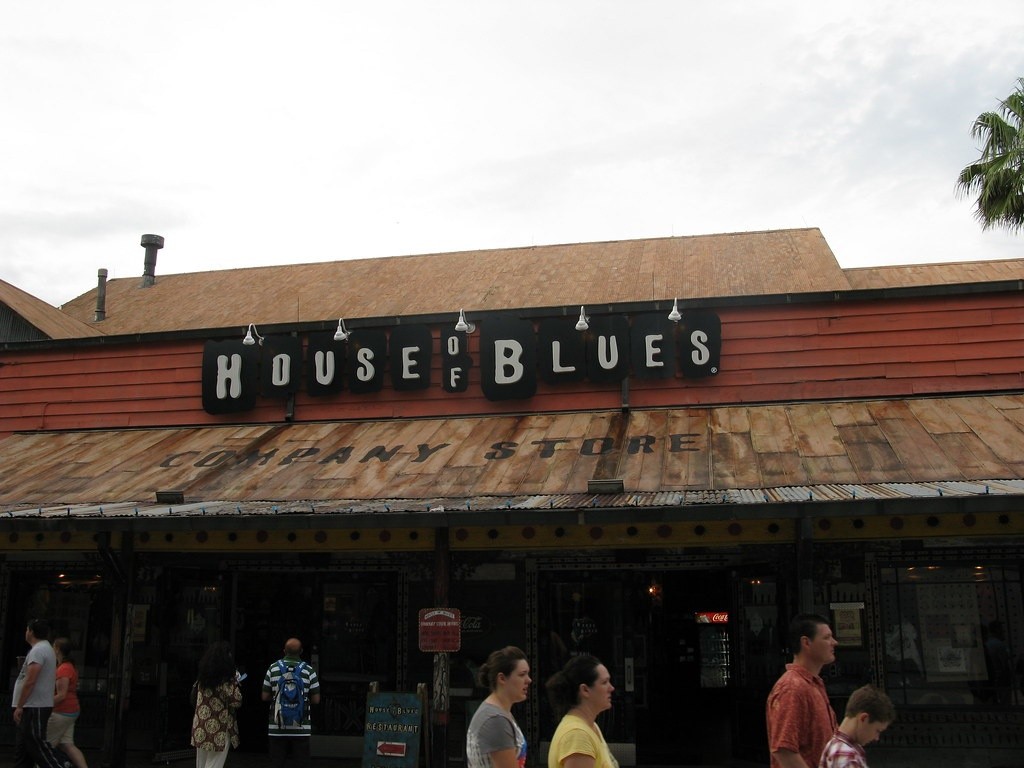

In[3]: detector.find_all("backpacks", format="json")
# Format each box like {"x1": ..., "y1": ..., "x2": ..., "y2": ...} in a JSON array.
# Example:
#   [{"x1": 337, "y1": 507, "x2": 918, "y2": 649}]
[{"x1": 274, "y1": 660, "x2": 306, "y2": 729}]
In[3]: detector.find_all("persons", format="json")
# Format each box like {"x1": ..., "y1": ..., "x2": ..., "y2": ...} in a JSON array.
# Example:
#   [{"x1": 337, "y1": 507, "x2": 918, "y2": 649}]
[
  {"x1": 966, "y1": 624, "x2": 995, "y2": 707},
  {"x1": 262, "y1": 638, "x2": 320, "y2": 768},
  {"x1": 190, "y1": 642, "x2": 242, "y2": 768},
  {"x1": 985, "y1": 620, "x2": 1012, "y2": 704},
  {"x1": 766, "y1": 613, "x2": 839, "y2": 768},
  {"x1": 541, "y1": 648, "x2": 620, "y2": 768},
  {"x1": 46, "y1": 638, "x2": 88, "y2": 768},
  {"x1": 818, "y1": 684, "x2": 896, "y2": 768},
  {"x1": 465, "y1": 646, "x2": 532, "y2": 768},
  {"x1": 11, "y1": 618, "x2": 66, "y2": 768}
]
